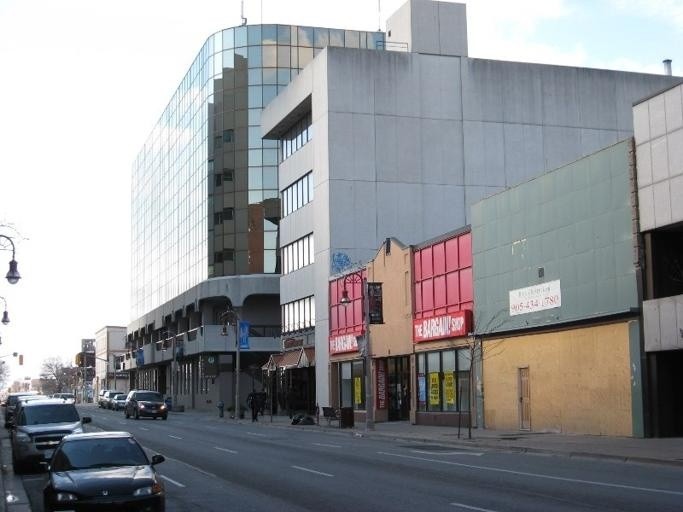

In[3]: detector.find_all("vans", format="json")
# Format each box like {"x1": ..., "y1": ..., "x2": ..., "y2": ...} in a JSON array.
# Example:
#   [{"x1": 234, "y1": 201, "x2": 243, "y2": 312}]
[
  {"x1": 98, "y1": 390, "x2": 113, "y2": 407},
  {"x1": 102, "y1": 391, "x2": 123, "y2": 410}
]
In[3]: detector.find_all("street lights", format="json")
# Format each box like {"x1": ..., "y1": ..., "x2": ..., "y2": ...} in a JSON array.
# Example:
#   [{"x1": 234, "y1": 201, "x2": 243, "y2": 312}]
[
  {"x1": 0, "y1": 294, "x2": 11, "y2": 328},
  {"x1": 340, "y1": 273, "x2": 375, "y2": 432},
  {"x1": 219, "y1": 308, "x2": 242, "y2": 420},
  {"x1": 160, "y1": 331, "x2": 177, "y2": 411},
  {"x1": 0, "y1": 233, "x2": 22, "y2": 285}
]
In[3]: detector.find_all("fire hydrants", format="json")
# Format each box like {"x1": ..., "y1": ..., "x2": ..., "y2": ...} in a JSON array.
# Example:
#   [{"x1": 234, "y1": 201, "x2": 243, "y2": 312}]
[{"x1": 215, "y1": 398, "x2": 224, "y2": 418}]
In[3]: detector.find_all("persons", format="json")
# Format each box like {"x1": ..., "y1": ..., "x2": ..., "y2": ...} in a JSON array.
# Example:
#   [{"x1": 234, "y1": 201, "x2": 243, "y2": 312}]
[
  {"x1": 257, "y1": 387, "x2": 268, "y2": 416},
  {"x1": 246, "y1": 388, "x2": 261, "y2": 423}
]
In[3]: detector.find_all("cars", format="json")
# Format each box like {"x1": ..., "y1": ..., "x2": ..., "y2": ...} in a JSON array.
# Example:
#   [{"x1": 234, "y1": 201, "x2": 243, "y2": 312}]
[
  {"x1": 39, "y1": 431, "x2": 167, "y2": 512},
  {"x1": 111, "y1": 394, "x2": 127, "y2": 411}
]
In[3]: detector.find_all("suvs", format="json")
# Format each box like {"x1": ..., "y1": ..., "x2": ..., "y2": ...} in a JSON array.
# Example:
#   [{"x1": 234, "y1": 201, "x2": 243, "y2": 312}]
[
  {"x1": 1, "y1": 391, "x2": 92, "y2": 473},
  {"x1": 123, "y1": 390, "x2": 167, "y2": 421}
]
